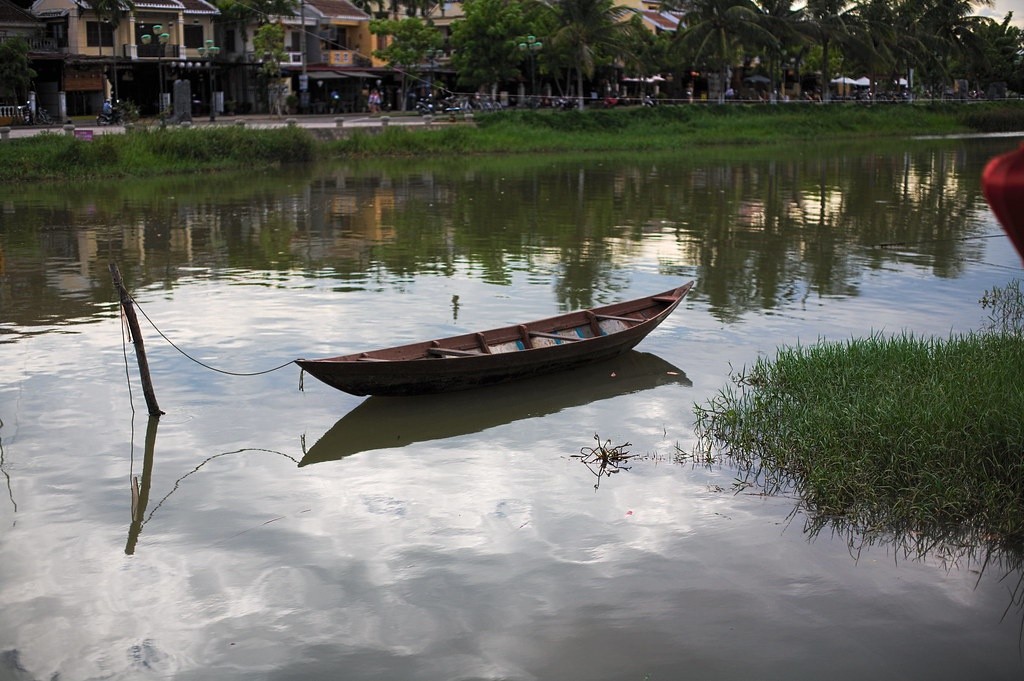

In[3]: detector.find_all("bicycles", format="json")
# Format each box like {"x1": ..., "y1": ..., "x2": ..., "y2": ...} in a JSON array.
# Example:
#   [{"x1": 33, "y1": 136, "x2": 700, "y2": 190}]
[{"x1": 35, "y1": 107, "x2": 54, "y2": 125}]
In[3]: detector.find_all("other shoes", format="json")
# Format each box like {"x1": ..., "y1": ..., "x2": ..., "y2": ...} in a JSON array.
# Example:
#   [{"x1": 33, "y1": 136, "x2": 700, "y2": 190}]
[{"x1": 107, "y1": 118, "x2": 111, "y2": 120}]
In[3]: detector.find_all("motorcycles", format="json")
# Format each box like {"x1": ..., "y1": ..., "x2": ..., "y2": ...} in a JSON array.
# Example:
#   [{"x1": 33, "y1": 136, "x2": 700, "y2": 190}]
[
  {"x1": 96, "y1": 108, "x2": 123, "y2": 126},
  {"x1": 17, "y1": 100, "x2": 34, "y2": 125}
]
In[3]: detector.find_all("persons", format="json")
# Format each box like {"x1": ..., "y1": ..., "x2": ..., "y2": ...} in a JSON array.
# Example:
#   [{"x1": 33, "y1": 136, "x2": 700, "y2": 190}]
[
  {"x1": 852, "y1": 88, "x2": 933, "y2": 100},
  {"x1": 287, "y1": 86, "x2": 494, "y2": 114},
  {"x1": 602, "y1": 92, "x2": 668, "y2": 107},
  {"x1": 724, "y1": 86, "x2": 822, "y2": 104},
  {"x1": 102, "y1": 99, "x2": 116, "y2": 119}
]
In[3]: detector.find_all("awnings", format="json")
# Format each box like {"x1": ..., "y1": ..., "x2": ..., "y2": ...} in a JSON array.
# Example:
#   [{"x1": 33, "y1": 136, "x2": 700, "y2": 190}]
[
  {"x1": 622, "y1": 75, "x2": 666, "y2": 83},
  {"x1": 306, "y1": 71, "x2": 382, "y2": 78}
]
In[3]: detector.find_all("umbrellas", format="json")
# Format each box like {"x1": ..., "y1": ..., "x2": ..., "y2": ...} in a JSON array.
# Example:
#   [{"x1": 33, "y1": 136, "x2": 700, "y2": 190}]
[
  {"x1": 743, "y1": 76, "x2": 771, "y2": 83},
  {"x1": 830, "y1": 76, "x2": 878, "y2": 86},
  {"x1": 894, "y1": 78, "x2": 908, "y2": 85}
]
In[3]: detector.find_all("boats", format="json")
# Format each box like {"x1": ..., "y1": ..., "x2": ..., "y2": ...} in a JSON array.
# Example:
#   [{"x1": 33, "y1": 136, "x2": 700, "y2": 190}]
[
  {"x1": 297, "y1": 352, "x2": 693, "y2": 471},
  {"x1": 292, "y1": 279, "x2": 695, "y2": 400}
]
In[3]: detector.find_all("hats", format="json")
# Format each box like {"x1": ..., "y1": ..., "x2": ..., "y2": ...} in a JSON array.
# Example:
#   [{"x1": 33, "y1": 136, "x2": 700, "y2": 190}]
[{"x1": 106, "y1": 99, "x2": 110, "y2": 101}]
